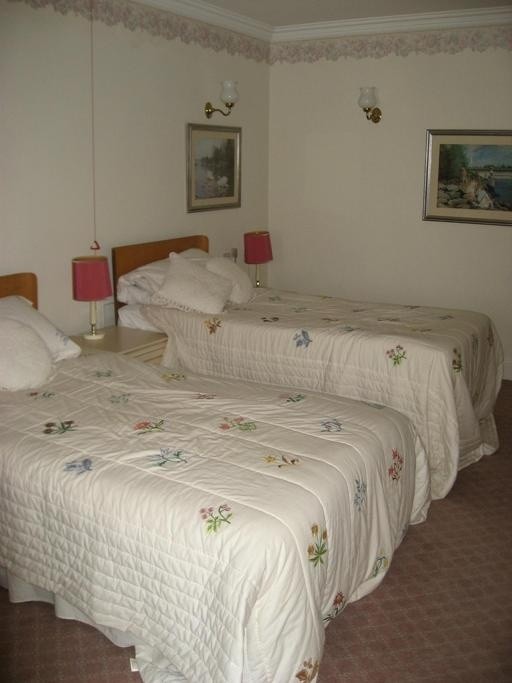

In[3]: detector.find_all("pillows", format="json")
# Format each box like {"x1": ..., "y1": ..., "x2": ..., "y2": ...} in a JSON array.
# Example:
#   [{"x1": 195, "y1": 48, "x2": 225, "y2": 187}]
[
  {"x1": 155, "y1": 252, "x2": 231, "y2": 315},
  {"x1": 0, "y1": 295, "x2": 80, "y2": 362},
  {"x1": 207, "y1": 258, "x2": 253, "y2": 303},
  {"x1": 115, "y1": 248, "x2": 211, "y2": 312},
  {"x1": 0, "y1": 317, "x2": 56, "y2": 393}
]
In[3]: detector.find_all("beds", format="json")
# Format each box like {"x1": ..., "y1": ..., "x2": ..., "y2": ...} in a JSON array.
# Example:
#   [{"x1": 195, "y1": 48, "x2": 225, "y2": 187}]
[
  {"x1": 111, "y1": 234, "x2": 506, "y2": 498},
  {"x1": 1, "y1": 273, "x2": 430, "y2": 683}
]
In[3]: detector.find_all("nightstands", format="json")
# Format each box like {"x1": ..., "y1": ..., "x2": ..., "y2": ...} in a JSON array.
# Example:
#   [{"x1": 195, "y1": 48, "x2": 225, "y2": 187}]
[{"x1": 68, "y1": 327, "x2": 168, "y2": 369}]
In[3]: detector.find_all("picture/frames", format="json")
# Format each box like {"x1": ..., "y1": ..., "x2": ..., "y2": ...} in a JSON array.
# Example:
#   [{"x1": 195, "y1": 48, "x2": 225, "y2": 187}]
[
  {"x1": 186, "y1": 122, "x2": 241, "y2": 212},
  {"x1": 421, "y1": 129, "x2": 512, "y2": 226}
]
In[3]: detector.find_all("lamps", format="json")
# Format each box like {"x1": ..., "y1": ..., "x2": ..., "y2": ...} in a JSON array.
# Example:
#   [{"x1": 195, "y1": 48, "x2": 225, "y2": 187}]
[
  {"x1": 205, "y1": 80, "x2": 239, "y2": 119},
  {"x1": 244, "y1": 230, "x2": 272, "y2": 288},
  {"x1": 72, "y1": 257, "x2": 112, "y2": 340},
  {"x1": 360, "y1": 87, "x2": 382, "y2": 123}
]
